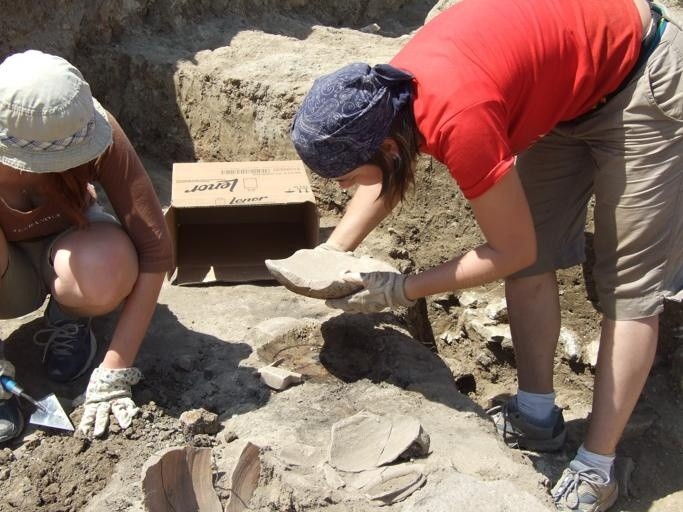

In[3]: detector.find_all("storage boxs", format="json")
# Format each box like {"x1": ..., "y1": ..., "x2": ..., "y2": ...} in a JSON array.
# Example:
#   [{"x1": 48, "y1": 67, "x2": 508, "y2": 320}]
[{"x1": 164, "y1": 160, "x2": 320, "y2": 286}]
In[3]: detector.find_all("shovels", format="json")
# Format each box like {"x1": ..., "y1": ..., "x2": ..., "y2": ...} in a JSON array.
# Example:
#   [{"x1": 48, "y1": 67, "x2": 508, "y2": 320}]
[{"x1": 0, "y1": 374, "x2": 75, "y2": 431}]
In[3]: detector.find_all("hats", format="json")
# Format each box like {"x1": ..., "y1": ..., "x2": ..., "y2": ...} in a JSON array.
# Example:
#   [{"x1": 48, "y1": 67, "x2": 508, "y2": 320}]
[
  {"x1": 323, "y1": 269, "x2": 417, "y2": 317},
  {"x1": 0, "y1": 47, "x2": 113, "y2": 177}
]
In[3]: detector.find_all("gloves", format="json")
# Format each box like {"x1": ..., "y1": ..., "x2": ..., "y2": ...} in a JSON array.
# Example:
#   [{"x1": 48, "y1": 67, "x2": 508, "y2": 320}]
[
  {"x1": 0, "y1": 358, "x2": 18, "y2": 400},
  {"x1": 76, "y1": 365, "x2": 143, "y2": 437}
]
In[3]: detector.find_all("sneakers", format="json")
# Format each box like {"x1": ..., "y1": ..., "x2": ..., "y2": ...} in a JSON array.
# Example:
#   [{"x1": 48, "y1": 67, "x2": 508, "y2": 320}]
[
  {"x1": 483, "y1": 395, "x2": 569, "y2": 453},
  {"x1": 0, "y1": 388, "x2": 26, "y2": 444},
  {"x1": 36, "y1": 293, "x2": 98, "y2": 384},
  {"x1": 550, "y1": 468, "x2": 618, "y2": 512}
]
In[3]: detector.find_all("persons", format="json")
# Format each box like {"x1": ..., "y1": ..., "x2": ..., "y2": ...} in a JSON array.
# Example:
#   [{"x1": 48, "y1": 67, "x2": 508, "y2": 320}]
[
  {"x1": 289, "y1": 1, "x2": 683, "y2": 511},
  {"x1": 0, "y1": 50, "x2": 174, "y2": 444}
]
